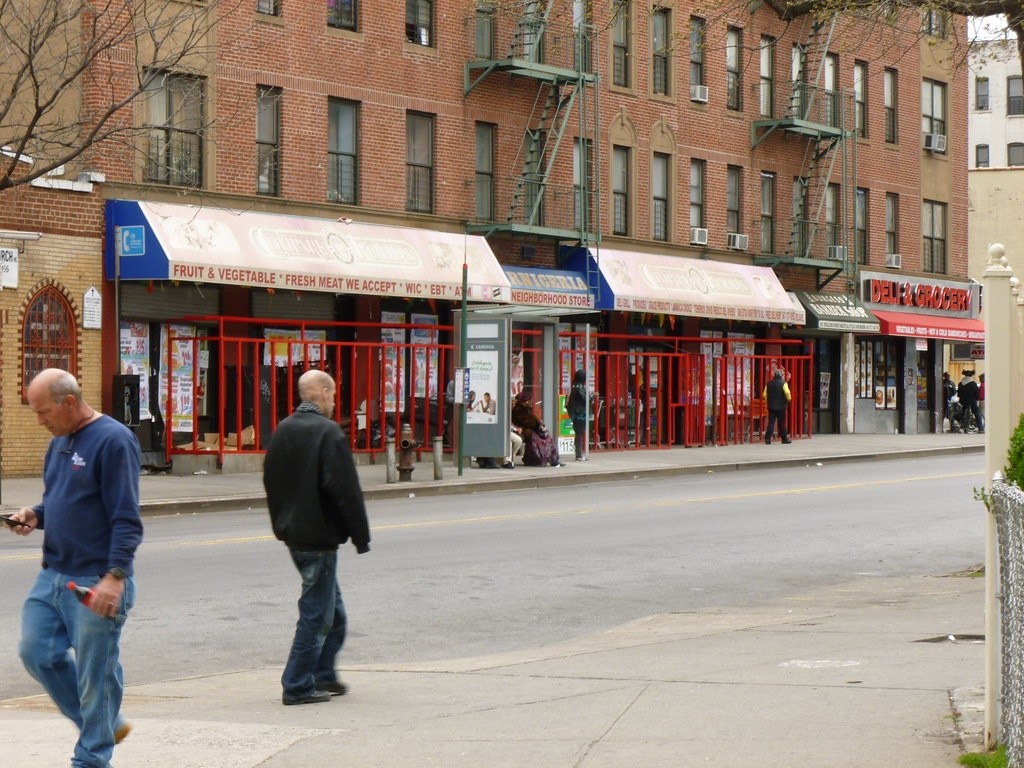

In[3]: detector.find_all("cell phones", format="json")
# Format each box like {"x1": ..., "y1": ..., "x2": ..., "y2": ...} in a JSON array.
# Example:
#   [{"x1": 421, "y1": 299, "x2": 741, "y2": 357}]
[{"x1": 0, "y1": 515, "x2": 31, "y2": 528}]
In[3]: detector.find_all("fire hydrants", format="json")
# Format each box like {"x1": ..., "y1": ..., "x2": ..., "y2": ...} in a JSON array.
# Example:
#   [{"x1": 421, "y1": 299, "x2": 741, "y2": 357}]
[{"x1": 395, "y1": 423, "x2": 416, "y2": 482}]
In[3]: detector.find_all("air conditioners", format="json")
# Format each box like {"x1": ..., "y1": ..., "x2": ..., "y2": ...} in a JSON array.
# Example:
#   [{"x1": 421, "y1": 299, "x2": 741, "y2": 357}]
[
  {"x1": 886, "y1": 253, "x2": 901, "y2": 268},
  {"x1": 689, "y1": 227, "x2": 708, "y2": 245},
  {"x1": 726, "y1": 233, "x2": 749, "y2": 250},
  {"x1": 827, "y1": 246, "x2": 847, "y2": 260},
  {"x1": 924, "y1": 133, "x2": 947, "y2": 151},
  {"x1": 689, "y1": 84, "x2": 709, "y2": 103}
]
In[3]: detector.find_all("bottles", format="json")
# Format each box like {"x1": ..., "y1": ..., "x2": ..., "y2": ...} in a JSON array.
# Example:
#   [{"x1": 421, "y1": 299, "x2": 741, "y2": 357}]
[{"x1": 65, "y1": 581, "x2": 118, "y2": 620}]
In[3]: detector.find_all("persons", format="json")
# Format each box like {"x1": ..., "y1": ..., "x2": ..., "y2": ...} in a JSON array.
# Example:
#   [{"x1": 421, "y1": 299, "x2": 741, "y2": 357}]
[
  {"x1": 762, "y1": 368, "x2": 792, "y2": 444},
  {"x1": 446, "y1": 379, "x2": 455, "y2": 403},
  {"x1": 978, "y1": 373, "x2": 985, "y2": 420},
  {"x1": 479, "y1": 392, "x2": 496, "y2": 415},
  {"x1": 466, "y1": 390, "x2": 479, "y2": 412},
  {"x1": 942, "y1": 372, "x2": 962, "y2": 433},
  {"x1": 564, "y1": 369, "x2": 600, "y2": 462},
  {"x1": 476, "y1": 456, "x2": 501, "y2": 468},
  {"x1": 6, "y1": 367, "x2": 143, "y2": 768},
  {"x1": 504, "y1": 425, "x2": 523, "y2": 469},
  {"x1": 958, "y1": 370, "x2": 984, "y2": 433},
  {"x1": 355, "y1": 397, "x2": 368, "y2": 449},
  {"x1": 511, "y1": 391, "x2": 566, "y2": 467},
  {"x1": 263, "y1": 369, "x2": 371, "y2": 706}
]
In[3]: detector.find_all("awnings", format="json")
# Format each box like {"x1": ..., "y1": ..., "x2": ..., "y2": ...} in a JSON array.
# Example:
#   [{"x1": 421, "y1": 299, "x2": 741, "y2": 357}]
[
  {"x1": 105, "y1": 198, "x2": 511, "y2": 305},
  {"x1": 870, "y1": 310, "x2": 985, "y2": 342},
  {"x1": 790, "y1": 287, "x2": 880, "y2": 333},
  {"x1": 560, "y1": 246, "x2": 796, "y2": 325}
]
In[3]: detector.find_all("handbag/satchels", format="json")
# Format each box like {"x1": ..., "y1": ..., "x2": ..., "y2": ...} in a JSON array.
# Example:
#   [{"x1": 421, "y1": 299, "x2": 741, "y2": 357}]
[{"x1": 535, "y1": 426, "x2": 549, "y2": 438}]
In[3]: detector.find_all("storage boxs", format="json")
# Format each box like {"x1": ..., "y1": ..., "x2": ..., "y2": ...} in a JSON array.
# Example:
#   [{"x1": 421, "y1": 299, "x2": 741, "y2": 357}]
[{"x1": 175, "y1": 423, "x2": 256, "y2": 451}]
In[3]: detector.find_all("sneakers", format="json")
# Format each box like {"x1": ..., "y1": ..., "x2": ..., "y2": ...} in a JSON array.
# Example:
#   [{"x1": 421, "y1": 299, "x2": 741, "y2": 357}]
[
  {"x1": 314, "y1": 680, "x2": 349, "y2": 693},
  {"x1": 282, "y1": 689, "x2": 330, "y2": 705}
]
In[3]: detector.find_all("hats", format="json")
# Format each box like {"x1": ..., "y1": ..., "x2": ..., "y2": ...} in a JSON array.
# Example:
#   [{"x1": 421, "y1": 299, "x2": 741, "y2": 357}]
[{"x1": 515, "y1": 392, "x2": 530, "y2": 402}]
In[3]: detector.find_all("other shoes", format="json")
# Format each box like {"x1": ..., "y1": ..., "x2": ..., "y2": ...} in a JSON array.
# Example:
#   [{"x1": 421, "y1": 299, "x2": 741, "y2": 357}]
[
  {"x1": 576, "y1": 456, "x2": 588, "y2": 462},
  {"x1": 765, "y1": 436, "x2": 771, "y2": 444},
  {"x1": 115, "y1": 723, "x2": 132, "y2": 744},
  {"x1": 979, "y1": 430, "x2": 985, "y2": 433},
  {"x1": 502, "y1": 462, "x2": 514, "y2": 468},
  {"x1": 551, "y1": 462, "x2": 565, "y2": 467},
  {"x1": 782, "y1": 438, "x2": 792, "y2": 444}
]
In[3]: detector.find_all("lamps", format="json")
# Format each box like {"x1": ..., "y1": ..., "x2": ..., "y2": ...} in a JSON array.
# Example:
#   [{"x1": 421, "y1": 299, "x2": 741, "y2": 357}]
[{"x1": 0, "y1": 229, "x2": 43, "y2": 253}]
